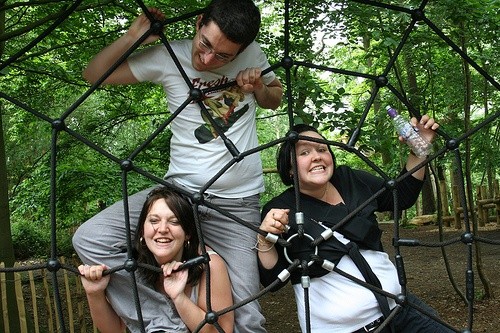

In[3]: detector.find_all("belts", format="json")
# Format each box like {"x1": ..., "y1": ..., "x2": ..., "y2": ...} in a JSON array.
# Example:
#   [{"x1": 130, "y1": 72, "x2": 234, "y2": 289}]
[{"x1": 352, "y1": 314, "x2": 386, "y2": 333}]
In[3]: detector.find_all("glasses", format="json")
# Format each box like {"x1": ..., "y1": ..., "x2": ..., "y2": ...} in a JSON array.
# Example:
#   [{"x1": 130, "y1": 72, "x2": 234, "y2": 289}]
[{"x1": 198, "y1": 32, "x2": 235, "y2": 64}]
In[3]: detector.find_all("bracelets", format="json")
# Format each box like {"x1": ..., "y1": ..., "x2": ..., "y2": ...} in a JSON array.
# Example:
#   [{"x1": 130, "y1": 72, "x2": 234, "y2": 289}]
[{"x1": 255, "y1": 239, "x2": 274, "y2": 252}]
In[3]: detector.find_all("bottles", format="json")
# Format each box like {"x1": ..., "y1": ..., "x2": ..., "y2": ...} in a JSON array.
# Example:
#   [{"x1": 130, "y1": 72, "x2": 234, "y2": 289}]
[{"x1": 385, "y1": 105, "x2": 432, "y2": 158}]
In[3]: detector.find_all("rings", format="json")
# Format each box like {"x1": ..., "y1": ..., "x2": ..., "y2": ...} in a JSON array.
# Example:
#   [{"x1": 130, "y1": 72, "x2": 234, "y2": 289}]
[{"x1": 272, "y1": 219, "x2": 276, "y2": 227}]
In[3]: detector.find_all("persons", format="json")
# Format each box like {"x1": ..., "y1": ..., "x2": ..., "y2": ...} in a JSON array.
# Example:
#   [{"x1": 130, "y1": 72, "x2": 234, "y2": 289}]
[
  {"x1": 78, "y1": 186, "x2": 234, "y2": 333},
  {"x1": 73, "y1": 0, "x2": 281, "y2": 333},
  {"x1": 257, "y1": 116, "x2": 463, "y2": 333}
]
